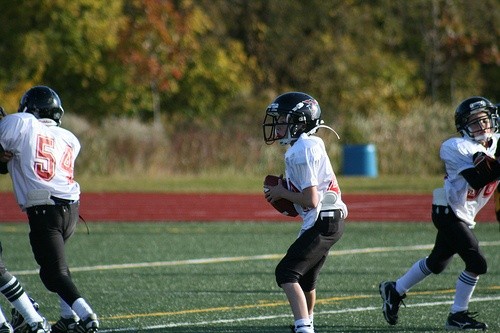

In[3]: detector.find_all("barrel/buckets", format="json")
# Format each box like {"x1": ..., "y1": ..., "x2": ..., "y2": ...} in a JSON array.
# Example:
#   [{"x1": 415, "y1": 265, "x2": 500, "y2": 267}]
[{"x1": 343, "y1": 144, "x2": 377, "y2": 177}]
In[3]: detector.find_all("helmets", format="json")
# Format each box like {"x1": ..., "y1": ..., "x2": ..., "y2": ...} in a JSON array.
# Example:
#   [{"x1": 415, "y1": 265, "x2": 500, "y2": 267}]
[
  {"x1": 454, "y1": 96, "x2": 500, "y2": 133},
  {"x1": 19, "y1": 85, "x2": 64, "y2": 122},
  {"x1": 266, "y1": 91, "x2": 321, "y2": 146}
]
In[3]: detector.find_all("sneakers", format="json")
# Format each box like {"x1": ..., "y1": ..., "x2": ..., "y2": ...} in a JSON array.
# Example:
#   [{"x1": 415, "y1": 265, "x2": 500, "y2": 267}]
[
  {"x1": 10, "y1": 297, "x2": 39, "y2": 330},
  {"x1": 68, "y1": 312, "x2": 99, "y2": 333},
  {"x1": 379, "y1": 280, "x2": 407, "y2": 326},
  {"x1": 0, "y1": 321, "x2": 14, "y2": 333},
  {"x1": 52, "y1": 316, "x2": 77, "y2": 333},
  {"x1": 19, "y1": 316, "x2": 52, "y2": 333},
  {"x1": 445, "y1": 310, "x2": 488, "y2": 333}
]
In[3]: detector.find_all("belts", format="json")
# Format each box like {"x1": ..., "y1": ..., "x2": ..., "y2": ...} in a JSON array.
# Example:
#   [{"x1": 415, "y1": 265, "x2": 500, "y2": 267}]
[
  {"x1": 320, "y1": 211, "x2": 343, "y2": 220},
  {"x1": 54, "y1": 198, "x2": 79, "y2": 206}
]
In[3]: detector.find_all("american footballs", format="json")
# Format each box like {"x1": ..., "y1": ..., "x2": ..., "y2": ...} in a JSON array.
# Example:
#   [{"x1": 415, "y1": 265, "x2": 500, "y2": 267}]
[{"x1": 264, "y1": 175, "x2": 300, "y2": 218}]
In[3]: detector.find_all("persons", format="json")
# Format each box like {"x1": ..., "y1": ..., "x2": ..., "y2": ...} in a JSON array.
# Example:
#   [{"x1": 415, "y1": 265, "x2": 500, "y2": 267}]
[
  {"x1": 0, "y1": 242, "x2": 53, "y2": 333},
  {"x1": 378, "y1": 96, "x2": 500, "y2": 331},
  {"x1": 0, "y1": 86, "x2": 99, "y2": 333},
  {"x1": 262, "y1": 92, "x2": 348, "y2": 333}
]
